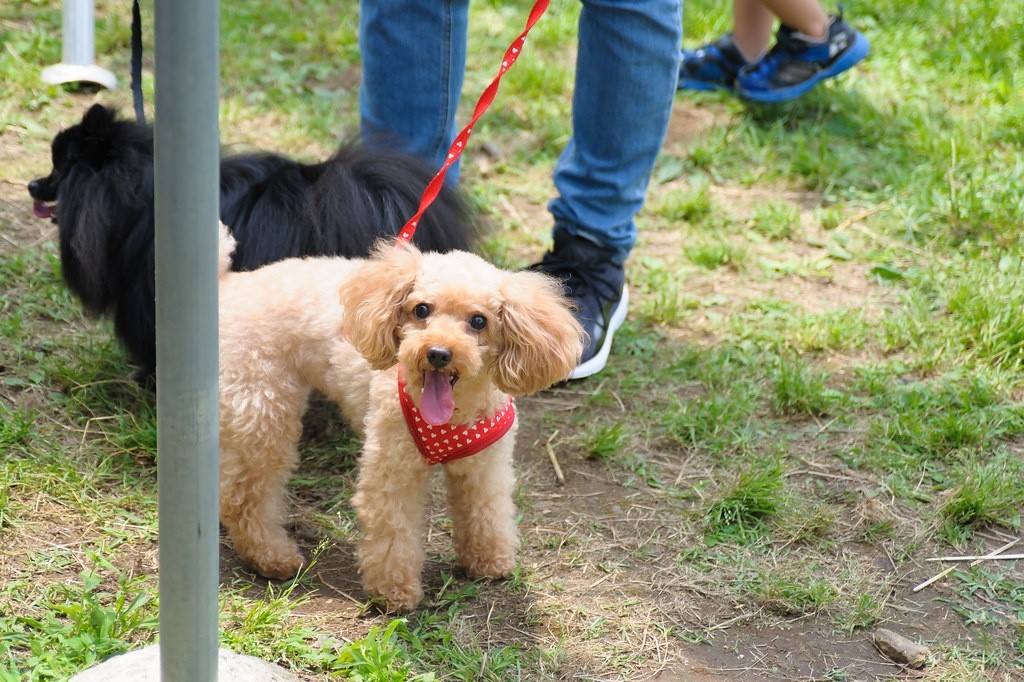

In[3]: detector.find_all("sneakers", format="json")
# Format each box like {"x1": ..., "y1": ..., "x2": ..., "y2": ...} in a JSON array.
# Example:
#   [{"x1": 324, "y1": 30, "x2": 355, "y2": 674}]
[
  {"x1": 735, "y1": 4, "x2": 870, "y2": 102},
  {"x1": 521, "y1": 223, "x2": 631, "y2": 379},
  {"x1": 676, "y1": 35, "x2": 751, "y2": 92}
]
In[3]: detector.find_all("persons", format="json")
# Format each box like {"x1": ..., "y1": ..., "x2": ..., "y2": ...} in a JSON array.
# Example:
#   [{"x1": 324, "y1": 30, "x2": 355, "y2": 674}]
[
  {"x1": 358, "y1": 1, "x2": 682, "y2": 380},
  {"x1": 677, "y1": 0, "x2": 869, "y2": 102}
]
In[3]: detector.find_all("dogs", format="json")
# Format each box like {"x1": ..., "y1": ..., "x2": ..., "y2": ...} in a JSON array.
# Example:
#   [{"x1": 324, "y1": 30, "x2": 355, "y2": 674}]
[
  {"x1": 27, "y1": 102, "x2": 481, "y2": 393},
  {"x1": 218, "y1": 214, "x2": 586, "y2": 617}
]
